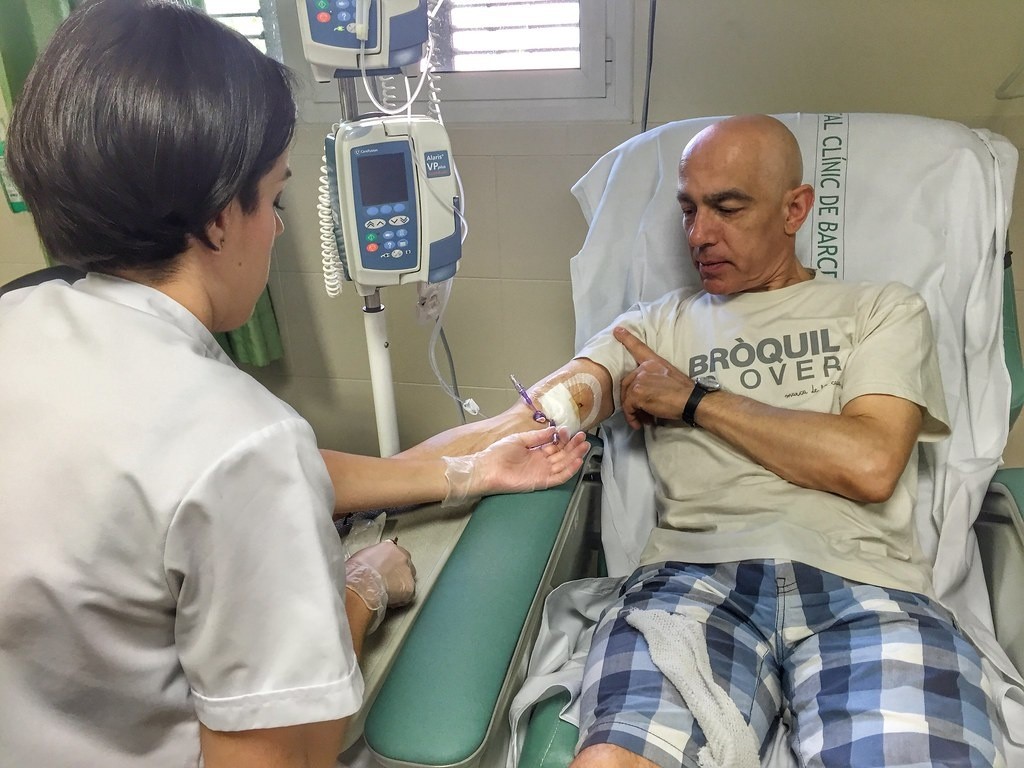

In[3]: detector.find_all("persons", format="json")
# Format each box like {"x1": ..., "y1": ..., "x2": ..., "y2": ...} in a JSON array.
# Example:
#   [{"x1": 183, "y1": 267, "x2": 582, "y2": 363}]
[
  {"x1": 386, "y1": 113, "x2": 995, "y2": 768},
  {"x1": 0, "y1": 0, "x2": 591, "y2": 768}
]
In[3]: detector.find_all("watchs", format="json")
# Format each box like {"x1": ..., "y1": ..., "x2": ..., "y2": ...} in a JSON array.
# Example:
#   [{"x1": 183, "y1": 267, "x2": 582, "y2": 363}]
[{"x1": 682, "y1": 373, "x2": 721, "y2": 427}]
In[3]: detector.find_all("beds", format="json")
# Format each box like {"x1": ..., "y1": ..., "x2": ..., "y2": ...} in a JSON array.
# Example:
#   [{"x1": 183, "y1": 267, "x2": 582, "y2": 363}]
[{"x1": 360, "y1": 113, "x2": 1024, "y2": 768}]
